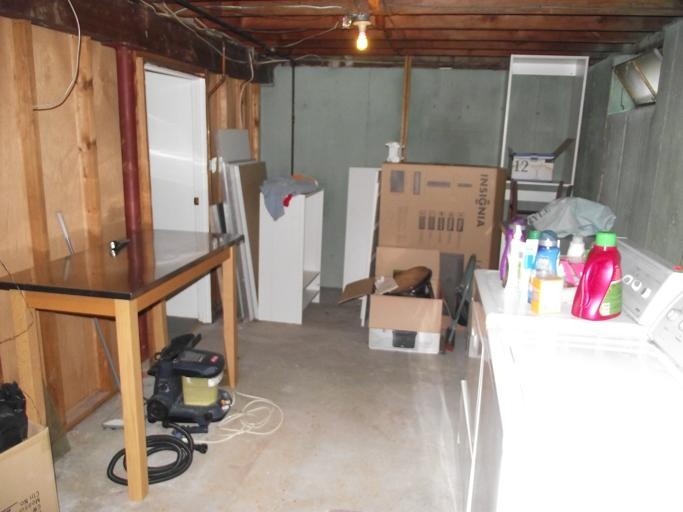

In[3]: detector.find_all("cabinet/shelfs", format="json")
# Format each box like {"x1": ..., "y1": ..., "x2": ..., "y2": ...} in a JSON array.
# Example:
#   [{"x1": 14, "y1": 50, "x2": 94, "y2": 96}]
[
  {"x1": 499, "y1": 53, "x2": 590, "y2": 204},
  {"x1": 259, "y1": 186, "x2": 324, "y2": 325}
]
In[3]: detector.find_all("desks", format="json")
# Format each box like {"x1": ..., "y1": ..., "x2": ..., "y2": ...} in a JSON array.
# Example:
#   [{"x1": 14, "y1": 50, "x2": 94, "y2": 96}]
[{"x1": 0, "y1": 229, "x2": 244, "y2": 502}]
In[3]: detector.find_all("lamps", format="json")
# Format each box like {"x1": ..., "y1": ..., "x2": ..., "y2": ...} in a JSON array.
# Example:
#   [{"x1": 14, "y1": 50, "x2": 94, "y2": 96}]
[{"x1": 352, "y1": 14, "x2": 372, "y2": 51}]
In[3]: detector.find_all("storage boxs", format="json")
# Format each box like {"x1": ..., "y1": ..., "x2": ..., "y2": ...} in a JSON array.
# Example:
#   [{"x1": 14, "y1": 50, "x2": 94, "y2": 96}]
[
  {"x1": 377, "y1": 163, "x2": 507, "y2": 269},
  {"x1": 510, "y1": 153, "x2": 555, "y2": 182},
  {"x1": 0, "y1": 417, "x2": 60, "y2": 512},
  {"x1": 337, "y1": 246, "x2": 452, "y2": 355}
]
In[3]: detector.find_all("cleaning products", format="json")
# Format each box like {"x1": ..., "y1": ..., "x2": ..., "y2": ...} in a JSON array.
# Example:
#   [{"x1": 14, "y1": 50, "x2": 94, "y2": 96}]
[{"x1": 570, "y1": 231, "x2": 624, "y2": 321}]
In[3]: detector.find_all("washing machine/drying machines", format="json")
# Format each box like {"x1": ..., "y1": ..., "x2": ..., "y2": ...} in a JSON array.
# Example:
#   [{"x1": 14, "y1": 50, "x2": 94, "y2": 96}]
[{"x1": 450, "y1": 234, "x2": 683, "y2": 512}]
